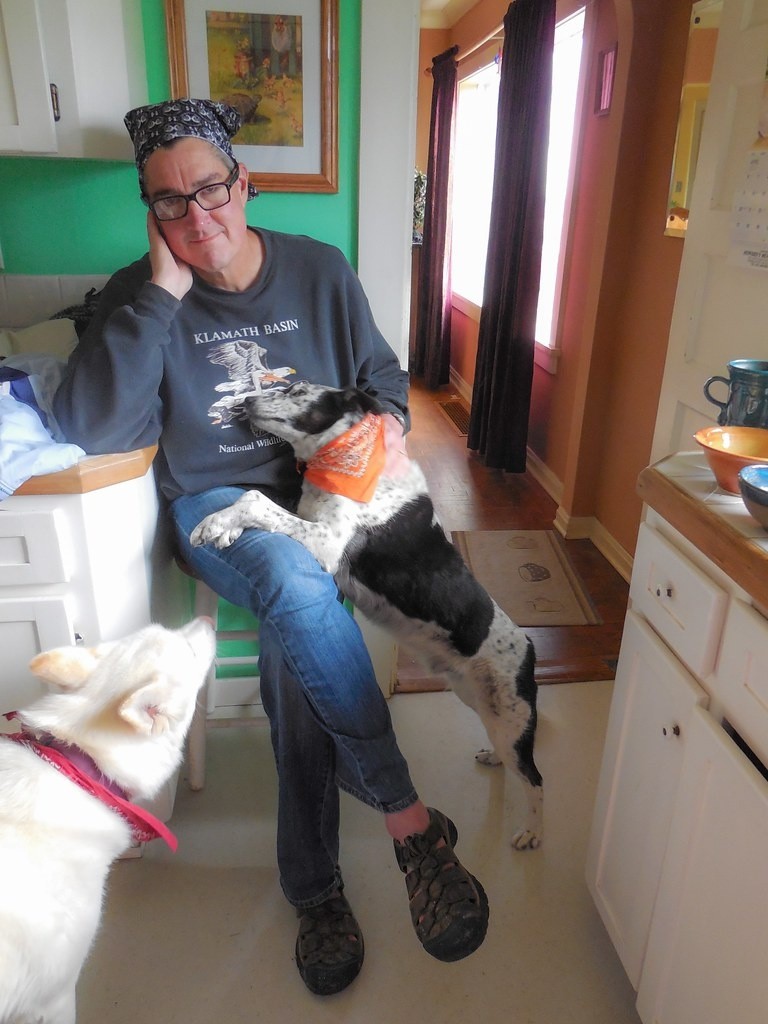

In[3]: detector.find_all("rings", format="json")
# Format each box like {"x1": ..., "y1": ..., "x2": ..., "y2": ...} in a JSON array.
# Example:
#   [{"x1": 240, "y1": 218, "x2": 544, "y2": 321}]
[{"x1": 398, "y1": 450, "x2": 404, "y2": 455}]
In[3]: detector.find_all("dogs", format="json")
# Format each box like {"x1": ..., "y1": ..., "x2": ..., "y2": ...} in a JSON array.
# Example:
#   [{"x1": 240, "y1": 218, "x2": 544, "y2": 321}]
[
  {"x1": 189, "y1": 381, "x2": 543, "y2": 849},
  {"x1": 0, "y1": 615, "x2": 217, "y2": 1024}
]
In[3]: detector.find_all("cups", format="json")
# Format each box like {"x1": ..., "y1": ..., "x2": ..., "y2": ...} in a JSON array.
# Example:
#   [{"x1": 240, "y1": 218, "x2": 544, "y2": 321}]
[{"x1": 703, "y1": 359, "x2": 768, "y2": 429}]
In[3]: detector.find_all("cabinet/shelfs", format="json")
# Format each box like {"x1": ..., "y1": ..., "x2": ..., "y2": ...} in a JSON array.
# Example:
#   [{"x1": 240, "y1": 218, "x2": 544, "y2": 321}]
[
  {"x1": 0, "y1": 0, "x2": 148, "y2": 161},
  {"x1": 582, "y1": 499, "x2": 768, "y2": 1024}
]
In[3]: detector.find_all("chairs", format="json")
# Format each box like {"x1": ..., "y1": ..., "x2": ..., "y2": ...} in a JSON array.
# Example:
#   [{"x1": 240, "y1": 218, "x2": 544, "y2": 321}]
[{"x1": 163, "y1": 547, "x2": 355, "y2": 788}]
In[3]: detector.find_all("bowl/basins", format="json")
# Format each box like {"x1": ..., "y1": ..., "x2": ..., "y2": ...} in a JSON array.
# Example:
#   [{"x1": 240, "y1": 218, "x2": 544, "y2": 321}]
[
  {"x1": 738, "y1": 464, "x2": 768, "y2": 533},
  {"x1": 694, "y1": 425, "x2": 768, "y2": 494}
]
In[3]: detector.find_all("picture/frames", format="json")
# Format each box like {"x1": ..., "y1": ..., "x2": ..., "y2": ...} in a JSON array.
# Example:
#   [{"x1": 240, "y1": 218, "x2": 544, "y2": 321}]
[{"x1": 163, "y1": 0, "x2": 341, "y2": 194}]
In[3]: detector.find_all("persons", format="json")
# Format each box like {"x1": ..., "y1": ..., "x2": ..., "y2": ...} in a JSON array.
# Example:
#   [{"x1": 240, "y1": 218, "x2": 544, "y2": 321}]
[{"x1": 52, "y1": 99, "x2": 491, "y2": 998}]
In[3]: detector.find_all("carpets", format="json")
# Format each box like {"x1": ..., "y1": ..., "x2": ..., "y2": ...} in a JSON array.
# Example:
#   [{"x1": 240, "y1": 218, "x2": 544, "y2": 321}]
[{"x1": 451, "y1": 528, "x2": 603, "y2": 627}]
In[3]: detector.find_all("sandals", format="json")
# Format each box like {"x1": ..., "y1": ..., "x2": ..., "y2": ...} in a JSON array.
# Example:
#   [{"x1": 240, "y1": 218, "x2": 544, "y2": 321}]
[
  {"x1": 292, "y1": 882, "x2": 369, "y2": 1000},
  {"x1": 387, "y1": 806, "x2": 489, "y2": 962}
]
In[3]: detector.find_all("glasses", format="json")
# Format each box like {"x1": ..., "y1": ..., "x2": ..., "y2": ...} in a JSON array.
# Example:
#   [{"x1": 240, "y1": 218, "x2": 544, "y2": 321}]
[{"x1": 148, "y1": 165, "x2": 243, "y2": 222}]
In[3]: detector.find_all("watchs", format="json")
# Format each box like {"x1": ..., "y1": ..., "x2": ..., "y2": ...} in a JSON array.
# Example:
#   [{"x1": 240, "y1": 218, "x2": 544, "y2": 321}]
[{"x1": 392, "y1": 412, "x2": 406, "y2": 427}]
